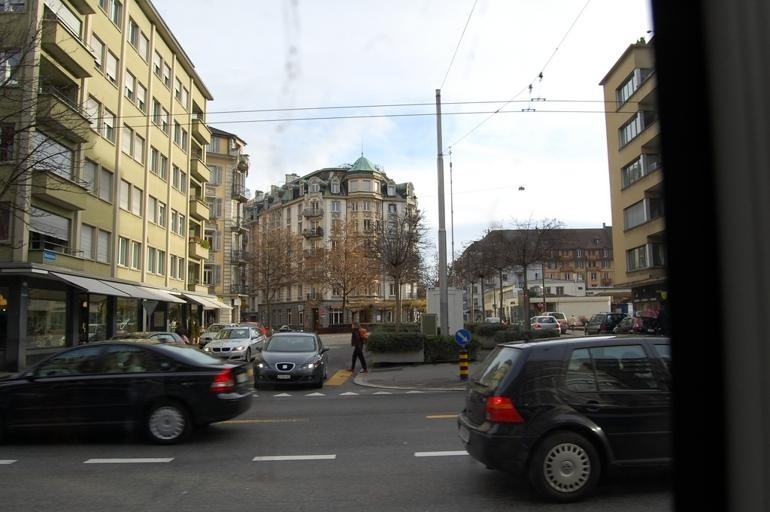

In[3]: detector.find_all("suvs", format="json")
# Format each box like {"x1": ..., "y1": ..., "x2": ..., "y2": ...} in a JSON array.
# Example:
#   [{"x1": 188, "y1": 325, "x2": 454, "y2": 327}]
[
  {"x1": 458, "y1": 335, "x2": 672, "y2": 503},
  {"x1": 583, "y1": 312, "x2": 627, "y2": 336},
  {"x1": 613, "y1": 316, "x2": 665, "y2": 336}
]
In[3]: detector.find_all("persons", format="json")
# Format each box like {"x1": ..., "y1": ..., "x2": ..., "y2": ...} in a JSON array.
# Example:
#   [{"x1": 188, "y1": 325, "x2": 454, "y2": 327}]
[
  {"x1": 569, "y1": 314, "x2": 576, "y2": 332},
  {"x1": 346, "y1": 320, "x2": 368, "y2": 373}
]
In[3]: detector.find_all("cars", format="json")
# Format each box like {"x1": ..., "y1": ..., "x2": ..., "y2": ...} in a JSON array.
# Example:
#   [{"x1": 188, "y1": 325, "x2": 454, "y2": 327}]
[
  {"x1": 530, "y1": 316, "x2": 561, "y2": 337},
  {"x1": 112, "y1": 331, "x2": 186, "y2": 344},
  {"x1": 61, "y1": 319, "x2": 138, "y2": 346},
  {"x1": 198, "y1": 320, "x2": 306, "y2": 364},
  {"x1": 0, "y1": 342, "x2": 253, "y2": 443},
  {"x1": 485, "y1": 317, "x2": 504, "y2": 324},
  {"x1": 253, "y1": 332, "x2": 331, "y2": 390}
]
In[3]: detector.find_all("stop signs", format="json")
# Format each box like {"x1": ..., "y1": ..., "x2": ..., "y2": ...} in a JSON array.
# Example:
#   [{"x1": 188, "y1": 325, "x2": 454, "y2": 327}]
[{"x1": 320, "y1": 311, "x2": 326, "y2": 317}]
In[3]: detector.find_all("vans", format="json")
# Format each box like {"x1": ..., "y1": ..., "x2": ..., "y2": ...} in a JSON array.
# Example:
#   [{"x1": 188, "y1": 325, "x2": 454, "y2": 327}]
[{"x1": 542, "y1": 312, "x2": 567, "y2": 333}]
